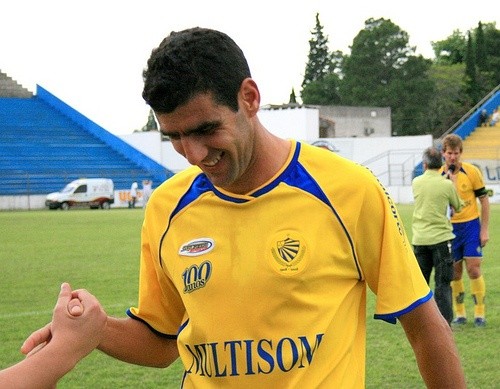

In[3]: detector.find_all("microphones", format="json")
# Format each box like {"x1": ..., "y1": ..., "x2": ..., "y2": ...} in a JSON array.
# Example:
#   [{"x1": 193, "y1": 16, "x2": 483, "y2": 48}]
[{"x1": 444, "y1": 164, "x2": 456, "y2": 179}]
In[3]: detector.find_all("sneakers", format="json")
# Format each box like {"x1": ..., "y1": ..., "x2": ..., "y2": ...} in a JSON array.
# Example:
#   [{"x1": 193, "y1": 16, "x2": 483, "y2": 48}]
[
  {"x1": 474, "y1": 316, "x2": 485, "y2": 327},
  {"x1": 453, "y1": 316, "x2": 467, "y2": 325}
]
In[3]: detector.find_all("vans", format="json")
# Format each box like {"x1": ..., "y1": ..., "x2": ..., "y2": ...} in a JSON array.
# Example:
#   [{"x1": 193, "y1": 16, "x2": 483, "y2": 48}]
[{"x1": 45, "y1": 178, "x2": 115, "y2": 210}]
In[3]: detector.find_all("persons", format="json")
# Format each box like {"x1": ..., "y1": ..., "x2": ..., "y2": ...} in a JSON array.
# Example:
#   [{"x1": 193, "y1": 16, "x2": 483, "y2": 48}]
[
  {"x1": 411, "y1": 147, "x2": 466, "y2": 331},
  {"x1": 127, "y1": 178, "x2": 152, "y2": 210},
  {"x1": 438, "y1": 134, "x2": 490, "y2": 327},
  {"x1": 0, "y1": 283, "x2": 108, "y2": 389},
  {"x1": 20, "y1": 26, "x2": 467, "y2": 389},
  {"x1": 480, "y1": 109, "x2": 500, "y2": 129}
]
